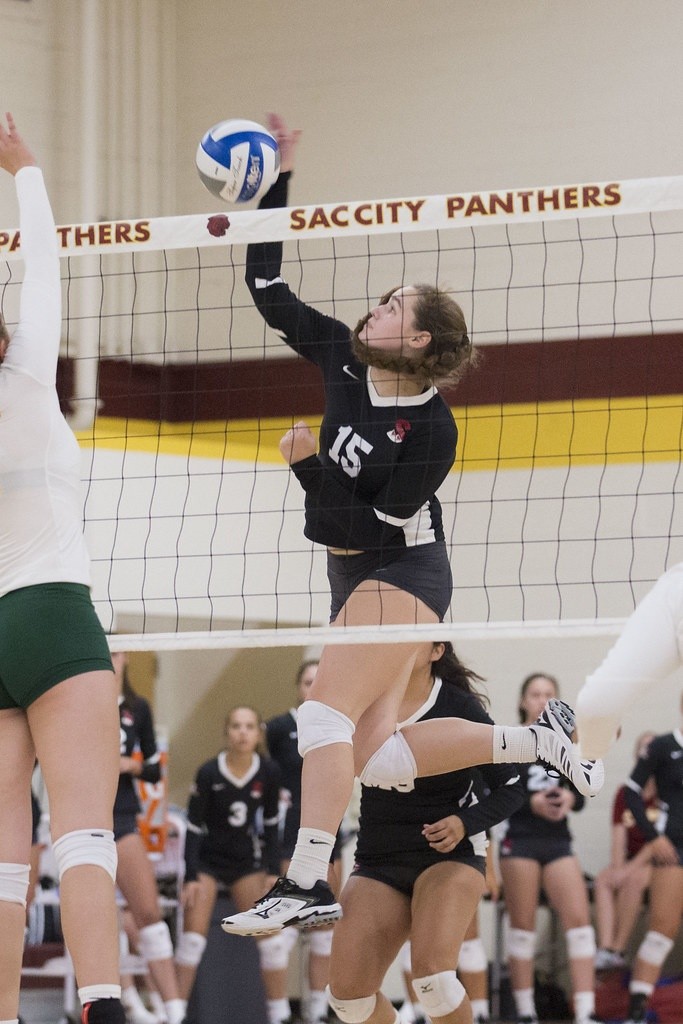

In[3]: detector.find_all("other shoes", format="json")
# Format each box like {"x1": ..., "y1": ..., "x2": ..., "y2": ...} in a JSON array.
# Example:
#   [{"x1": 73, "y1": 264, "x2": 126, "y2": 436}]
[
  {"x1": 575, "y1": 1012, "x2": 617, "y2": 1024},
  {"x1": 593, "y1": 945, "x2": 626, "y2": 969},
  {"x1": 626, "y1": 990, "x2": 649, "y2": 1024},
  {"x1": 519, "y1": 1012, "x2": 540, "y2": 1024}
]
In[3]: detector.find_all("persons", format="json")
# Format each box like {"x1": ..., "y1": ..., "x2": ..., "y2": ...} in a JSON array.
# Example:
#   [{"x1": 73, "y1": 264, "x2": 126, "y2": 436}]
[
  {"x1": 0, "y1": 110, "x2": 129, "y2": 1024},
  {"x1": 25, "y1": 561, "x2": 683, "y2": 1024},
  {"x1": 219, "y1": 113, "x2": 604, "y2": 938}
]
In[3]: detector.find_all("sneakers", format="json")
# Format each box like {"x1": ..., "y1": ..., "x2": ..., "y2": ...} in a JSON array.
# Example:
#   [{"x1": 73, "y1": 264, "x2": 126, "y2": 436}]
[
  {"x1": 221, "y1": 878, "x2": 343, "y2": 937},
  {"x1": 528, "y1": 699, "x2": 604, "y2": 799}
]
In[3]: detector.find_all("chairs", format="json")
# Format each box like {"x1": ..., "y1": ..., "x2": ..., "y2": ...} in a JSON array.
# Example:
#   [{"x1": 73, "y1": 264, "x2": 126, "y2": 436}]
[
  {"x1": 116, "y1": 813, "x2": 186, "y2": 952},
  {"x1": 28, "y1": 882, "x2": 59, "y2": 945}
]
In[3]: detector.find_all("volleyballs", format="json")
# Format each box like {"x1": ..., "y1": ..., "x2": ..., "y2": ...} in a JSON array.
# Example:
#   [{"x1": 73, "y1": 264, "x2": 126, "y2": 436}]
[{"x1": 195, "y1": 117, "x2": 282, "y2": 205}]
